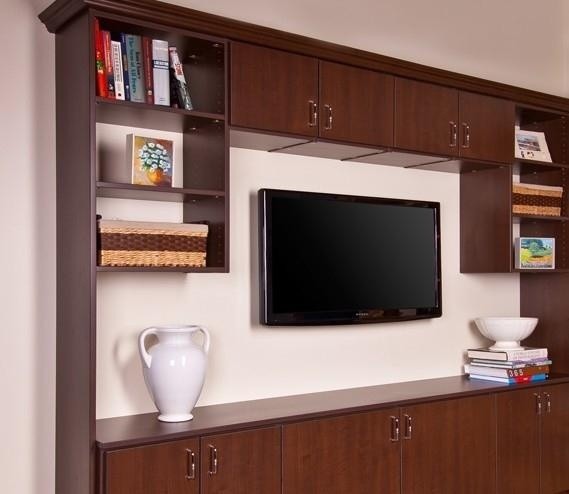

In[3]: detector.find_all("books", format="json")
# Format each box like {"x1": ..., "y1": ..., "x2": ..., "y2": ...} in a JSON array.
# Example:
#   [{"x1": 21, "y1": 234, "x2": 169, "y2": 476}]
[
  {"x1": 470, "y1": 357, "x2": 548, "y2": 365},
  {"x1": 92, "y1": 16, "x2": 195, "y2": 111},
  {"x1": 466, "y1": 345, "x2": 549, "y2": 360},
  {"x1": 469, "y1": 360, "x2": 554, "y2": 369},
  {"x1": 467, "y1": 372, "x2": 548, "y2": 384},
  {"x1": 464, "y1": 363, "x2": 550, "y2": 378}
]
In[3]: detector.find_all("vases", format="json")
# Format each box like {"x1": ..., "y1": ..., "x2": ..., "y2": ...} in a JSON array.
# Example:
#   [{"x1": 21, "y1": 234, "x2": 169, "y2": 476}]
[{"x1": 146, "y1": 168, "x2": 165, "y2": 184}]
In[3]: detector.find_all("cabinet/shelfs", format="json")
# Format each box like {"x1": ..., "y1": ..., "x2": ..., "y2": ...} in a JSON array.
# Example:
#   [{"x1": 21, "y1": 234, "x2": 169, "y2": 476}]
[
  {"x1": 497, "y1": 383, "x2": 569, "y2": 494},
  {"x1": 395, "y1": 58, "x2": 513, "y2": 173},
  {"x1": 39, "y1": 2, "x2": 230, "y2": 273},
  {"x1": 230, "y1": 17, "x2": 395, "y2": 162},
  {"x1": 282, "y1": 394, "x2": 497, "y2": 494},
  {"x1": 459, "y1": 73, "x2": 569, "y2": 273},
  {"x1": 96, "y1": 425, "x2": 282, "y2": 494}
]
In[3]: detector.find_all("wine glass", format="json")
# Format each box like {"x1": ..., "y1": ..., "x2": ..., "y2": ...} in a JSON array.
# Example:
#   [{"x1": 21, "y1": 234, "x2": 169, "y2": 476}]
[{"x1": 475, "y1": 316, "x2": 541, "y2": 352}]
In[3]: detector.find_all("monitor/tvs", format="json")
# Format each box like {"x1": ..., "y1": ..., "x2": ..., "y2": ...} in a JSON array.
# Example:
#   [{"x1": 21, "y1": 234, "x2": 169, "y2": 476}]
[{"x1": 257, "y1": 188, "x2": 442, "y2": 326}]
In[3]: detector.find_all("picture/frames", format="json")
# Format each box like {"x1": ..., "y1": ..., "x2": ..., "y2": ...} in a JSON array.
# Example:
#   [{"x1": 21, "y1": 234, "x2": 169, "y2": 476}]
[
  {"x1": 515, "y1": 237, "x2": 555, "y2": 270},
  {"x1": 126, "y1": 133, "x2": 176, "y2": 186}
]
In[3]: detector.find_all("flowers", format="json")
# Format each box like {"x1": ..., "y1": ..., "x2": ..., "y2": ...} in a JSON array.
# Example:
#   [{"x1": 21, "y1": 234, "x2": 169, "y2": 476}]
[{"x1": 138, "y1": 142, "x2": 171, "y2": 175}]
